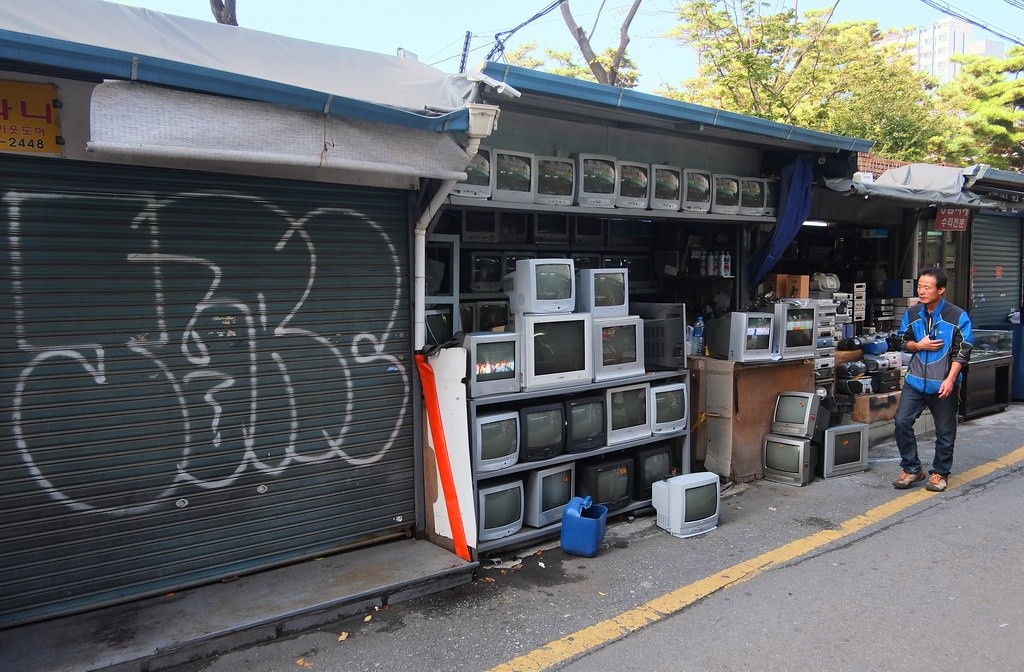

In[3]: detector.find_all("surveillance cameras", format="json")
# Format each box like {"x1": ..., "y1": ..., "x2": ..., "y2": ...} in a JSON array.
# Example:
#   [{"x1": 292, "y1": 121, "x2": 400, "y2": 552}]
[{"x1": 497, "y1": 82, "x2": 521, "y2": 100}]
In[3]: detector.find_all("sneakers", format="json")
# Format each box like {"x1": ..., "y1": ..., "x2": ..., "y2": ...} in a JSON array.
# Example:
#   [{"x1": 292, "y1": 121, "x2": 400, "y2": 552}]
[
  {"x1": 926, "y1": 472, "x2": 948, "y2": 492},
  {"x1": 892, "y1": 470, "x2": 926, "y2": 490}
]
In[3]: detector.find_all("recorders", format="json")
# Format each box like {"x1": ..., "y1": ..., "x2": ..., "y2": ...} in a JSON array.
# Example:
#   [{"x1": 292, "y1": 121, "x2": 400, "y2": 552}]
[
  {"x1": 835, "y1": 338, "x2": 902, "y2": 395},
  {"x1": 810, "y1": 272, "x2": 840, "y2": 291}
]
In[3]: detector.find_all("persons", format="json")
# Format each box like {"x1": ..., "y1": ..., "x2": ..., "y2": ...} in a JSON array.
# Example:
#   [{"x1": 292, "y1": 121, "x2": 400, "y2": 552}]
[{"x1": 891, "y1": 267, "x2": 973, "y2": 491}]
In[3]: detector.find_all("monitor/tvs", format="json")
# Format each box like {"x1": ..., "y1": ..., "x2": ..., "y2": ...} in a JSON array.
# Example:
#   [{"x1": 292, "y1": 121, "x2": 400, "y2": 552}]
[
  {"x1": 425, "y1": 147, "x2": 831, "y2": 541},
  {"x1": 816, "y1": 423, "x2": 869, "y2": 480}
]
